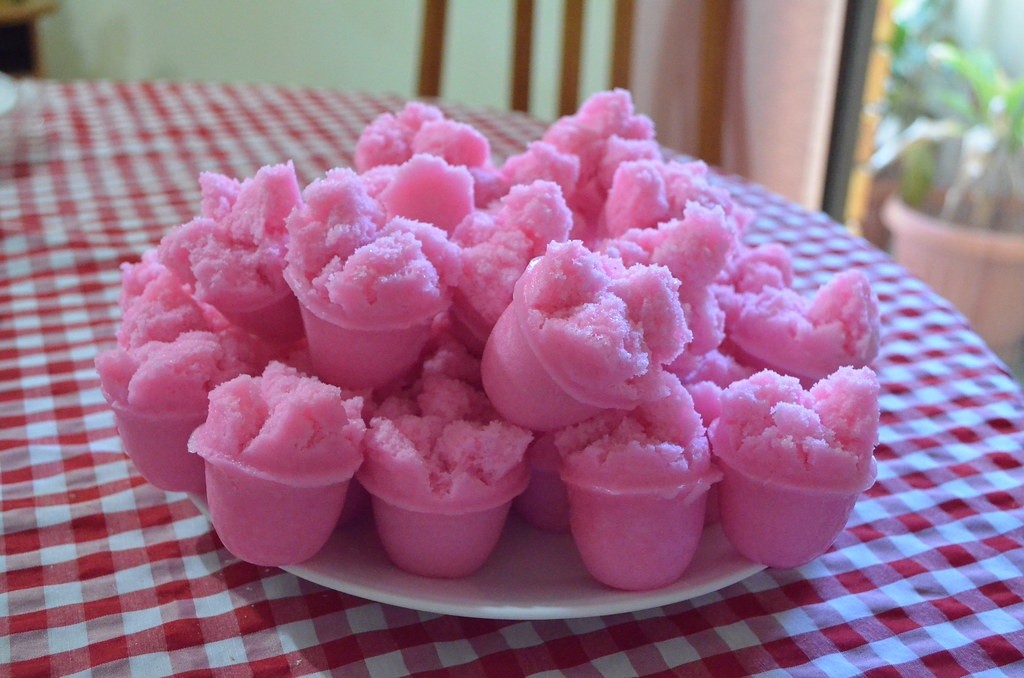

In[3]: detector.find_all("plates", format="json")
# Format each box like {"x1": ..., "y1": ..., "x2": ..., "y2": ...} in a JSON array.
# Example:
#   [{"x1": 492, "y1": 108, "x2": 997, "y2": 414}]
[{"x1": 185, "y1": 491, "x2": 771, "y2": 620}]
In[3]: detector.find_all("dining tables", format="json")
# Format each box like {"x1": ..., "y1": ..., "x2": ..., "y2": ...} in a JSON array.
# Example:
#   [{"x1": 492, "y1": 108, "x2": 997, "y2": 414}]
[{"x1": 0, "y1": 73, "x2": 1024, "y2": 678}]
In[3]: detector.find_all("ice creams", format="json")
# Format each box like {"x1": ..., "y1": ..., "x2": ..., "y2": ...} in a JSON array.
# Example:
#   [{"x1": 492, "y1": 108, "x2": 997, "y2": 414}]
[{"x1": 94, "y1": 88, "x2": 881, "y2": 591}]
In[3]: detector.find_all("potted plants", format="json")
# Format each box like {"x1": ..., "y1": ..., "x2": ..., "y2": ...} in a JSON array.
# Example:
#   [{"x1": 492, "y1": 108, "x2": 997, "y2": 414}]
[{"x1": 877, "y1": 30, "x2": 1024, "y2": 366}]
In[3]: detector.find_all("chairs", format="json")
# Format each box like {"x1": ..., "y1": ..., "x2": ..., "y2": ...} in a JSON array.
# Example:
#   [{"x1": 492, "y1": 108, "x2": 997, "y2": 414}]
[{"x1": 411, "y1": 0, "x2": 732, "y2": 171}]
